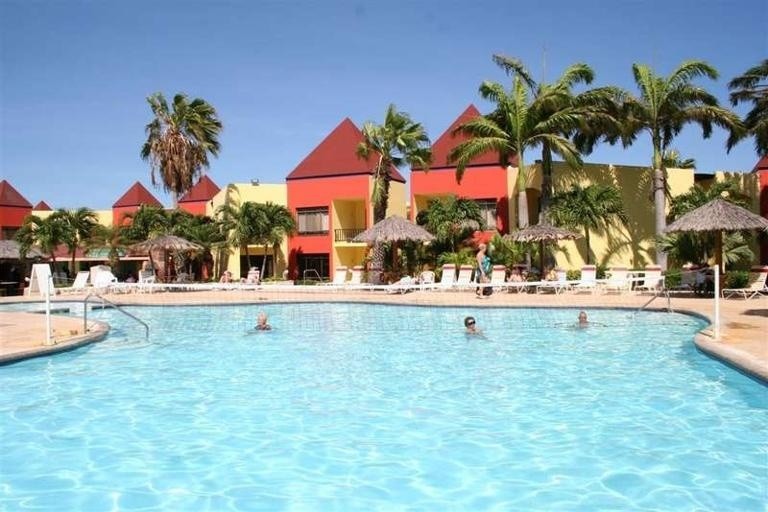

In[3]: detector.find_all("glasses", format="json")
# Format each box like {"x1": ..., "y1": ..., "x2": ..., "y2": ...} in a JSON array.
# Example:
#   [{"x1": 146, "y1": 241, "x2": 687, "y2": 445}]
[{"x1": 468, "y1": 320, "x2": 475, "y2": 325}]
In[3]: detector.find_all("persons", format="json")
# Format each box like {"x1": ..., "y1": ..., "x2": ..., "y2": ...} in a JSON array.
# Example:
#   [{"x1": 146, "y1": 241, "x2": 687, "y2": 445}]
[
  {"x1": 253, "y1": 312, "x2": 272, "y2": 331},
  {"x1": 462, "y1": 316, "x2": 481, "y2": 338},
  {"x1": 505, "y1": 264, "x2": 559, "y2": 293},
  {"x1": 165, "y1": 250, "x2": 215, "y2": 284},
  {"x1": 475, "y1": 243, "x2": 491, "y2": 299},
  {"x1": 577, "y1": 310, "x2": 587, "y2": 328}
]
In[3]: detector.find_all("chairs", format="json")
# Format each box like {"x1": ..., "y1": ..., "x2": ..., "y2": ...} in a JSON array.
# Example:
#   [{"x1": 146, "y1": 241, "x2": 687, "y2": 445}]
[
  {"x1": 433, "y1": 264, "x2": 664, "y2": 294},
  {"x1": 58, "y1": 271, "x2": 294, "y2": 295},
  {"x1": 316, "y1": 263, "x2": 364, "y2": 293},
  {"x1": 721, "y1": 265, "x2": 768, "y2": 301},
  {"x1": 366, "y1": 271, "x2": 435, "y2": 294}
]
